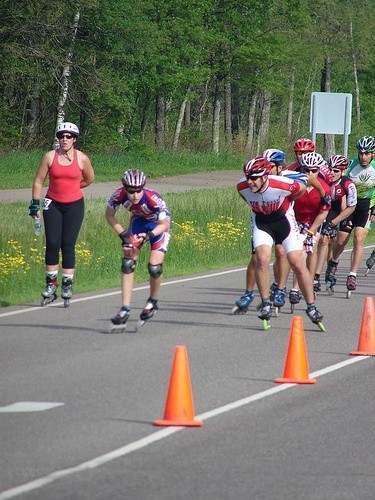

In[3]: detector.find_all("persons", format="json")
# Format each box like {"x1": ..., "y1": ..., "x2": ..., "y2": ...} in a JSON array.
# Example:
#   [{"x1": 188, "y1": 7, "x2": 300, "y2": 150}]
[
  {"x1": 29, "y1": 122, "x2": 95, "y2": 307},
  {"x1": 106, "y1": 169, "x2": 170, "y2": 332},
  {"x1": 237, "y1": 157, "x2": 325, "y2": 331},
  {"x1": 232, "y1": 136, "x2": 375, "y2": 316}
]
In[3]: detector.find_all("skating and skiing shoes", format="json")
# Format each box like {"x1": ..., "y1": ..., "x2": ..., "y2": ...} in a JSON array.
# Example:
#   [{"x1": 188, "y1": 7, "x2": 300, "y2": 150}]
[
  {"x1": 289, "y1": 289, "x2": 300, "y2": 313},
  {"x1": 61, "y1": 277, "x2": 74, "y2": 308},
  {"x1": 313, "y1": 259, "x2": 358, "y2": 299},
  {"x1": 269, "y1": 283, "x2": 278, "y2": 303},
  {"x1": 108, "y1": 306, "x2": 130, "y2": 332},
  {"x1": 306, "y1": 306, "x2": 325, "y2": 331},
  {"x1": 231, "y1": 292, "x2": 254, "y2": 314},
  {"x1": 364, "y1": 252, "x2": 375, "y2": 275},
  {"x1": 257, "y1": 300, "x2": 273, "y2": 330},
  {"x1": 139, "y1": 297, "x2": 159, "y2": 326},
  {"x1": 274, "y1": 290, "x2": 284, "y2": 317},
  {"x1": 40, "y1": 273, "x2": 58, "y2": 306}
]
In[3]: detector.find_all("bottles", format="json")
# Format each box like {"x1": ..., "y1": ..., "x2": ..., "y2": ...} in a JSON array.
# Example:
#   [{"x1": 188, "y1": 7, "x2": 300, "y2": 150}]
[{"x1": 34, "y1": 214, "x2": 42, "y2": 236}]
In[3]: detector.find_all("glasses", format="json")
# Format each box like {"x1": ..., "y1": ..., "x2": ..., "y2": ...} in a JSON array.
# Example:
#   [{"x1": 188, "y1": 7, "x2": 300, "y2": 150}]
[
  {"x1": 57, "y1": 134, "x2": 75, "y2": 139},
  {"x1": 303, "y1": 167, "x2": 318, "y2": 173},
  {"x1": 126, "y1": 188, "x2": 143, "y2": 194},
  {"x1": 270, "y1": 164, "x2": 276, "y2": 168},
  {"x1": 246, "y1": 175, "x2": 263, "y2": 180},
  {"x1": 329, "y1": 168, "x2": 341, "y2": 173}
]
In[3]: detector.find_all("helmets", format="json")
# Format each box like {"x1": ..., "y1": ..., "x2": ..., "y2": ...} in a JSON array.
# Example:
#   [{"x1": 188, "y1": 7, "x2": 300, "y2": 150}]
[
  {"x1": 243, "y1": 156, "x2": 272, "y2": 176},
  {"x1": 356, "y1": 136, "x2": 375, "y2": 153},
  {"x1": 262, "y1": 149, "x2": 285, "y2": 163},
  {"x1": 293, "y1": 139, "x2": 314, "y2": 152},
  {"x1": 121, "y1": 169, "x2": 146, "y2": 186},
  {"x1": 55, "y1": 121, "x2": 79, "y2": 138},
  {"x1": 301, "y1": 154, "x2": 349, "y2": 168}
]
